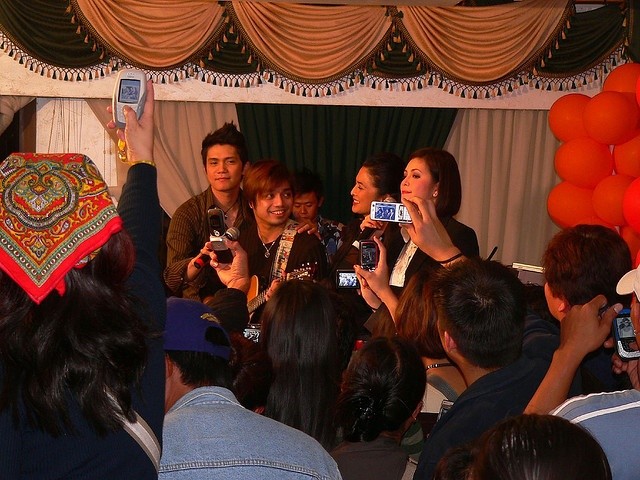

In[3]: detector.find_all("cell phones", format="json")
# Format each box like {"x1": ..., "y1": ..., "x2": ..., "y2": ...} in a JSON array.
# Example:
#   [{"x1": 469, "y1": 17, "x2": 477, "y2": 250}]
[
  {"x1": 336, "y1": 270, "x2": 360, "y2": 289},
  {"x1": 207, "y1": 209, "x2": 228, "y2": 250},
  {"x1": 360, "y1": 242, "x2": 379, "y2": 272},
  {"x1": 370, "y1": 202, "x2": 415, "y2": 224},
  {"x1": 614, "y1": 309, "x2": 640, "y2": 359},
  {"x1": 112, "y1": 68, "x2": 147, "y2": 130},
  {"x1": 243, "y1": 323, "x2": 261, "y2": 345},
  {"x1": 436, "y1": 400, "x2": 455, "y2": 425}
]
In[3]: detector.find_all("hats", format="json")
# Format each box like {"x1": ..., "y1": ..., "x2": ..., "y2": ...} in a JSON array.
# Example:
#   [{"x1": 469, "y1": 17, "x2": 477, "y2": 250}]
[
  {"x1": 616, "y1": 265, "x2": 640, "y2": 302},
  {"x1": 162, "y1": 298, "x2": 231, "y2": 359}
]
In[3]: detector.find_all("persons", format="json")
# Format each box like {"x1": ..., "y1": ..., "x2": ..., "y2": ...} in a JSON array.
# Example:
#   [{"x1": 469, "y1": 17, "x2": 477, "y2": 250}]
[
  {"x1": 328, "y1": 153, "x2": 401, "y2": 265},
  {"x1": 399, "y1": 195, "x2": 583, "y2": 480},
  {"x1": 353, "y1": 235, "x2": 467, "y2": 437},
  {"x1": 523, "y1": 262, "x2": 640, "y2": 480},
  {"x1": 330, "y1": 271, "x2": 426, "y2": 480},
  {"x1": 160, "y1": 120, "x2": 250, "y2": 295},
  {"x1": 289, "y1": 167, "x2": 346, "y2": 265},
  {"x1": 242, "y1": 278, "x2": 425, "y2": 462},
  {"x1": 545, "y1": 224, "x2": 633, "y2": 323},
  {"x1": 158, "y1": 297, "x2": 342, "y2": 480},
  {"x1": 183, "y1": 160, "x2": 327, "y2": 324},
  {"x1": 202, "y1": 237, "x2": 251, "y2": 333},
  {"x1": 0, "y1": 80, "x2": 167, "y2": 480},
  {"x1": 474, "y1": 414, "x2": 613, "y2": 480},
  {"x1": 432, "y1": 440, "x2": 474, "y2": 480},
  {"x1": 386, "y1": 146, "x2": 479, "y2": 295}
]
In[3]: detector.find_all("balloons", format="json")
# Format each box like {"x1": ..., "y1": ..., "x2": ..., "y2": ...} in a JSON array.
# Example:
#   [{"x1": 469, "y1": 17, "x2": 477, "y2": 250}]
[
  {"x1": 624, "y1": 177, "x2": 640, "y2": 234},
  {"x1": 612, "y1": 136, "x2": 640, "y2": 179},
  {"x1": 547, "y1": 181, "x2": 595, "y2": 229},
  {"x1": 604, "y1": 62, "x2": 640, "y2": 92},
  {"x1": 554, "y1": 140, "x2": 615, "y2": 189},
  {"x1": 549, "y1": 94, "x2": 589, "y2": 142},
  {"x1": 582, "y1": 89, "x2": 640, "y2": 144},
  {"x1": 593, "y1": 175, "x2": 638, "y2": 226},
  {"x1": 621, "y1": 223, "x2": 640, "y2": 263}
]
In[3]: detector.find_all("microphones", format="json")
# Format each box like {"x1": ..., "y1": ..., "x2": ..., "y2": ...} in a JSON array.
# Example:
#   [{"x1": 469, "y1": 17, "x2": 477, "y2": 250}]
[{"x1": 194, "y1": 227, "x2": 240, "y2": 269}]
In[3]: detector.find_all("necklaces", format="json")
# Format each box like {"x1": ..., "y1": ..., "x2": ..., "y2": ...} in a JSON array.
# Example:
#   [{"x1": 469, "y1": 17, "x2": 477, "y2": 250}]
[
  {"x1": 214, "y1": 193, "x2": 241, "y2": 221},
  {"x1": 425, "y1": 362, "x2": 457, "y2": 371},
  {"x1": 256, "y1": 225, "x2": 284, "y2": 259}
]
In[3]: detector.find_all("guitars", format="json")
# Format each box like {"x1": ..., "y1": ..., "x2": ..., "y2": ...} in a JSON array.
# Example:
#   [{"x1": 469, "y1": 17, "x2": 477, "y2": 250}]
[{"x1": 247, "y1": 262, "x2": 318, "y2": 324}]
